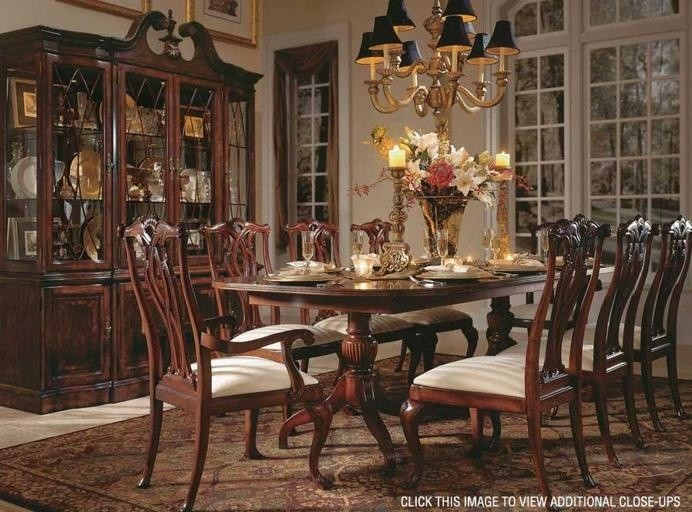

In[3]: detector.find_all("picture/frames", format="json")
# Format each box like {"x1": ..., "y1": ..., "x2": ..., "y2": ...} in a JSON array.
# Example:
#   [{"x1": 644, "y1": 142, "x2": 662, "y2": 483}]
[
  {"x1": 55, "y1": 0, "x2": 152, "y2": 22},
  {"x1": 186, "y1": 0, "x2": 263, "y2": 51},
  {"x1": 9, "y1": 76, "x2": 37, "y2": 129},
  {"x1": 178, "y1": 104, "x2": 212, "y2": 142}
]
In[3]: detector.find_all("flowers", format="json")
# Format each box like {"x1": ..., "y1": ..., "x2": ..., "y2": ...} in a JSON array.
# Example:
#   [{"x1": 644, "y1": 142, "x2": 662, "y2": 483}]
[{"x1": 346, "y1": 123, "x2": 534, "y2": 212}]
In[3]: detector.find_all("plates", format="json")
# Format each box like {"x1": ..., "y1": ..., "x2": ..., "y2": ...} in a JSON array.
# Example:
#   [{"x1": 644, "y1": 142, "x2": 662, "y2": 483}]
[{"x1": 4, "y1": 75, "x2": 242, "y2": 266}]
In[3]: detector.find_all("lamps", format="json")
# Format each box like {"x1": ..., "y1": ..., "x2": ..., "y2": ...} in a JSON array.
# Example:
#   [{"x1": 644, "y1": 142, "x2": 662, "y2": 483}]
[{"x1": 351, "y1": 0, "x2": 524, "y2": 119}]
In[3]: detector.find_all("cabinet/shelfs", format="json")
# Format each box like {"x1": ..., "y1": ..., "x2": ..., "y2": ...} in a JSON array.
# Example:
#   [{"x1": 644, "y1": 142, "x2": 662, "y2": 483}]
[
  {"x1": 0, "y1": 9, "x2": 266, "y2": 275},
  {"x1": 0, "y1": 276, "x2": 256, "y2": 415}
]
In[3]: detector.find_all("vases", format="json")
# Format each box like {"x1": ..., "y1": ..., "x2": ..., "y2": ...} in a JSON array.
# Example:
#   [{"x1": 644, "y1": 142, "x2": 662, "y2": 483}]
[{"x1": 412, "y1": 195, "x2": 471, "y2": 266}]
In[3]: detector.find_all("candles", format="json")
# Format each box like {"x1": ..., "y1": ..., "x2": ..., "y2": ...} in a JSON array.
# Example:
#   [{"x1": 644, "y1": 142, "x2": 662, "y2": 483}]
[
  {"x1": 494, "y1": 149, "x2": 512, "y2": 166},
  {"x1": 387, "y1": 144, "x2": 407, "y2": 168}
]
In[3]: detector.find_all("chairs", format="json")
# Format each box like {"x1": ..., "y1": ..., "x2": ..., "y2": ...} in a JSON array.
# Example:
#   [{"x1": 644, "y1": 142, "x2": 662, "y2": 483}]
[
  {"x1": 544, "y1": 213, "x2": 691, "y2": 431},
  {"x1": 347, "y1": 216, "x2": 482, "y2": 382},
  {"x1": 397, "y1": 211, "x2": 613, "y2": 512},
  {"x1": 113, "y1": 211, "x2": 340, "y2": 511},
  {"x1": 506, "y1": 218, "x2": 607, "y2": 422},
  {"x1": 280, "y1": 217, "x2": 425, "y2": 396},
  {"x1": 484, "y1": 212, "x2": 661, "y2": 469},
  {"x1": 196, "y1": 215, "x2": 364, "y2": 436}
]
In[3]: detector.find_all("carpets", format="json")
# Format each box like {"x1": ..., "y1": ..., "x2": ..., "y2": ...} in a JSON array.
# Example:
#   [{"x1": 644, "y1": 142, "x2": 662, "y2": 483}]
[{"x1": 0, "y1": 349, "x2": 691, "y2": 512}]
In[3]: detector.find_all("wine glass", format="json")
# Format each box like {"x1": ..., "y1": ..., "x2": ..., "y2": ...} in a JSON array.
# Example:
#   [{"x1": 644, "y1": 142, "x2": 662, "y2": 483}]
[{"x1": 264, "y1": 227, "x2": 603, "y2": 283}]
